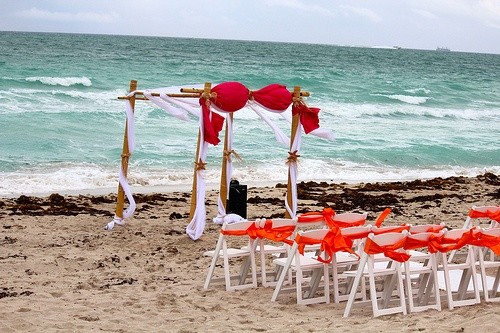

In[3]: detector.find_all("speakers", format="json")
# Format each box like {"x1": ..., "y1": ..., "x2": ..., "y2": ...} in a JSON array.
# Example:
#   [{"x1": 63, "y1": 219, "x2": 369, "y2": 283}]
[{"x1": 230, "y1": 185, "x2": 247, "y2": 218}]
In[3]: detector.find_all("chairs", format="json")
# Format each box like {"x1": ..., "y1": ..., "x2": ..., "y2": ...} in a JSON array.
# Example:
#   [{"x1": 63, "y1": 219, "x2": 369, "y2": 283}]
[{"x1": 202, "y1": 205, "x2": 500, "y2": 319}]
